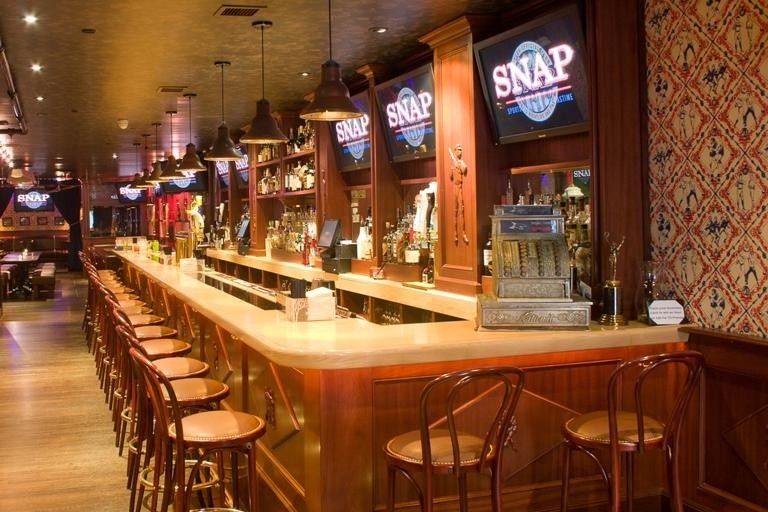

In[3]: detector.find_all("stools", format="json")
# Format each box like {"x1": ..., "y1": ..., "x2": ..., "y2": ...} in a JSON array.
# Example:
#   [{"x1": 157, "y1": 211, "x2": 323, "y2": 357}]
[
  {"x1": 111, "y1": 308, "x2": 213, "y2": 486},
  {"x1": 77, "y1": 250, "x2": 193, "y2": 456},
  {"x1": 114, "y1": 322, "x2": 229, "y2": 511},
  {"x1": 127, "y1": 346, "x2": 267, "y2": 512}
]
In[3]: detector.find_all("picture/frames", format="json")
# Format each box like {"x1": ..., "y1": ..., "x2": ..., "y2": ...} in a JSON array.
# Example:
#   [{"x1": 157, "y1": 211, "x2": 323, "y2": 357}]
[{"x1": 0, "y1": 214, "x2": 65, "y2": 227}]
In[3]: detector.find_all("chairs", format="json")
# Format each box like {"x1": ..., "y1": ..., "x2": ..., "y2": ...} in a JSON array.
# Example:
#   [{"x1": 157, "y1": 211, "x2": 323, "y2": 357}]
[
  {"x1": 556, "y1": 348, "x2": 707, "y2": 512},
  {"x1": 382, "y1": 362, "x2": 526, "y2": 511}
]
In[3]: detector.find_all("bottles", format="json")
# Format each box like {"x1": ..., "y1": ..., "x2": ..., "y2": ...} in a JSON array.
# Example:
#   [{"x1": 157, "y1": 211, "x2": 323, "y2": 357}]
[
  {"x1": 356, "y1": 205, "x2": 372, "y2": 259},
  {"x1": 482, "y1": 169, "x2": 590, "y2": 294},
  {"x1": 264, "y1": 206, "x2": 318, "y2": 268},
  {"x1": 638, "y1": 262, "x2": 666, "y2": 323},
  {"x1": 256, "y1": 119, "x2": 316, "y2": 195},
  {"x1": 380, "y1": 202, "x2": 434, "y2": 285}
]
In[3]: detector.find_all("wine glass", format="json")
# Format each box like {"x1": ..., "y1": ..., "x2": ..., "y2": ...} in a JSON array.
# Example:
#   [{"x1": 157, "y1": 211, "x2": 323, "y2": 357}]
[{"x1": 374, "y1": 299, "x2": 400, "y2": 325}]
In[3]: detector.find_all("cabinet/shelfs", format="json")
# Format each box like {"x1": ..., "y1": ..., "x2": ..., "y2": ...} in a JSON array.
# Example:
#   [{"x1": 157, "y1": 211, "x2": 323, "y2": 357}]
[
  {"x1": 251, "y1": 103, "x2": 320, "y2": 201},
  {"x1": 212, "y1": 136, "x2": 278, "y2": 257}
]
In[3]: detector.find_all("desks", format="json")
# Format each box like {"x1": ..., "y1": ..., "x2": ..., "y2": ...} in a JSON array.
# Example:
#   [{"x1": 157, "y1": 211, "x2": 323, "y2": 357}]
[{"x1": 0, "y1": 251, "x2": 44, "y2": 297}]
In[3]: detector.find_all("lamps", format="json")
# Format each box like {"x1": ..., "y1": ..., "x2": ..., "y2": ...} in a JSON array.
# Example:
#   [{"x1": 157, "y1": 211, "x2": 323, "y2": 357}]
[
  {"x1": 10, "y1": 159, "x2": 24, "y2": 179},
  {"x1": 238, "y1": 18, "x2": 291, "y2": 146},
  {"x1": 202, "y1": 59, "x2": 245, "y2": 164},
  {"x1": 128, "y1": 121, "x2": 170, "y2": 191},
  {"x1": 158, "y1": 109, "x2": 190, "y2": 182},
  {"x1": 297, "y1": 2, "x2": 364, "y2": 126},
  {"x1": 174, "y1": 92, "x2": 208, "y2": 174}
]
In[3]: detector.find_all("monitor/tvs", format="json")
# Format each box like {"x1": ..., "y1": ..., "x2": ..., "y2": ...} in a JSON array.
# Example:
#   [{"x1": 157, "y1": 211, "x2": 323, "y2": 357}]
[
  {"x1": 237, "y1": 219, "x2": 251, "y2": 237},
  {"x1": 317, "y1": 217, "x2": 342, "y2": 250}
]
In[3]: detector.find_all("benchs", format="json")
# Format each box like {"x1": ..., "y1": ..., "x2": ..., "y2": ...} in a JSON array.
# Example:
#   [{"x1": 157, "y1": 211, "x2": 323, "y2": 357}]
[
  {"x1": 29, "y1": 261, "x2": 57, "y2": 300},
  {"x1": 0, "y1": 262, "x2": 18, "y2": 301}
]
